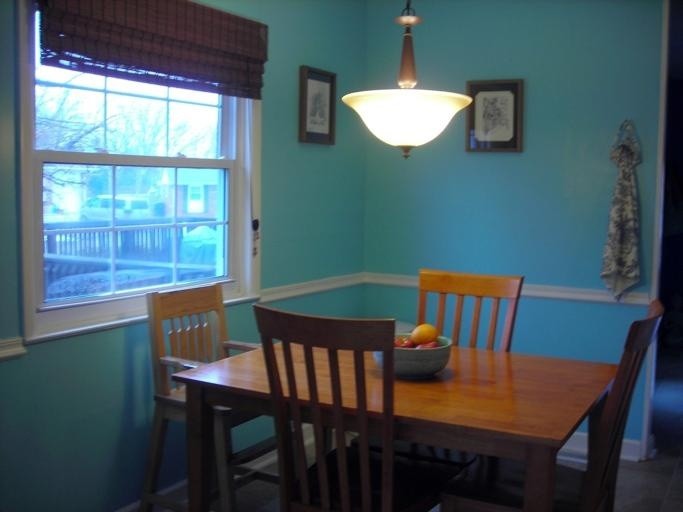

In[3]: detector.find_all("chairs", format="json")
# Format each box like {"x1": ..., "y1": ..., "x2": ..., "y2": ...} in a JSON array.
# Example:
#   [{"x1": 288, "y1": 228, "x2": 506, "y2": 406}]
[
  {"x1": 144, "y1": 283, "x2": 279, "y2": 510},
  {"x1": 253, "y1": 303, "x2": 396, "y2": 510},
  {"x1": 429, "y1": 316, "x2": 661, "y2": 512},
  {"x1": 349, "y1": 271, "x2": 525, "y2": 467}
]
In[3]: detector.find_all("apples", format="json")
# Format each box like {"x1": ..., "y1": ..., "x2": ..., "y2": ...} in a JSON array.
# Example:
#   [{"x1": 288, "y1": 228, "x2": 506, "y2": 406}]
[
  {"x1": 416, "y1": 341, "x2": 440, "y2": 349},
  {"x1": 394, "y1": 336, "x2": 413, "y2": 348}
]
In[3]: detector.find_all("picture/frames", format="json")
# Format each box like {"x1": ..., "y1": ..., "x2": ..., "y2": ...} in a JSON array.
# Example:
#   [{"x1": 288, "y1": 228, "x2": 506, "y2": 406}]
[
  {"x1": 298, "y1": 63, "x2": 335, "y2": 146},
  {"x1": 465, "y1": 78, "x2": 524, "y2": 154}
]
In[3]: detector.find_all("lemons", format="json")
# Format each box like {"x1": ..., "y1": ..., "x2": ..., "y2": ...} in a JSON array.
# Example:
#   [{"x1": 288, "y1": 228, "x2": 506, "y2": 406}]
[{"x1": 410, "y1": 324, "x2": 437, "y2": 345}]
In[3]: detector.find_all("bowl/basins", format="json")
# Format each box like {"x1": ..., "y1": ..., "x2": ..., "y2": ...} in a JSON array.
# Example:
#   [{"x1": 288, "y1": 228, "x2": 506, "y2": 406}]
[{"x1": 374, "y1": 331, "x2": 452, "y2": 381}]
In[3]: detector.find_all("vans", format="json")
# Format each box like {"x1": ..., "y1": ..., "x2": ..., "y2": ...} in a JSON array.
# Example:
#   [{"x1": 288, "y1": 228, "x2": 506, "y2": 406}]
[{"x1": 78, "y1": 194, "x2": 151, "y2": 222}]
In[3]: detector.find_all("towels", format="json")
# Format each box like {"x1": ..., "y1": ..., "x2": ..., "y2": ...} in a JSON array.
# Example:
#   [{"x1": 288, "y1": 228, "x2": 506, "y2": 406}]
[{"x1": 602, "y1": 120, "x2": 642, "y2": 299}]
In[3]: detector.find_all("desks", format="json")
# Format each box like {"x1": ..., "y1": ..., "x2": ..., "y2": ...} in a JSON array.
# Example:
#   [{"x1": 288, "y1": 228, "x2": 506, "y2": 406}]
[{"x1": 173, "y1": 339, "x2": 621, "y2": 511}]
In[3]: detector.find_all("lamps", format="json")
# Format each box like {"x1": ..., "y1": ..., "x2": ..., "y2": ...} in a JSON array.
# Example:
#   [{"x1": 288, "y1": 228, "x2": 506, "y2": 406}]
[{"x1": 341, "y1": 0, "x2": 473, "y2": 160}]
